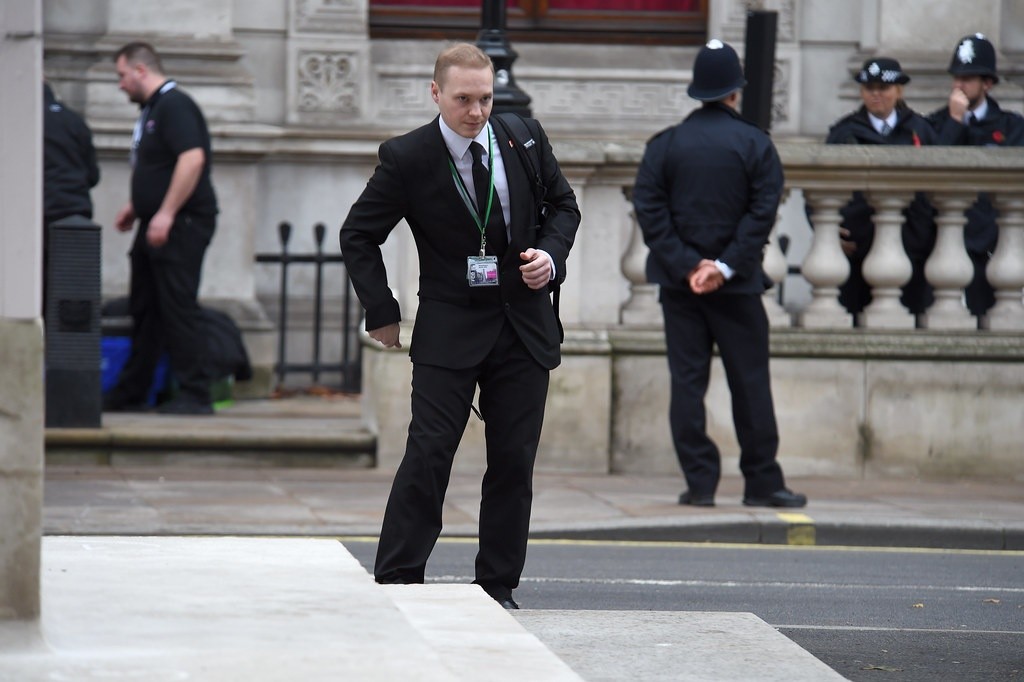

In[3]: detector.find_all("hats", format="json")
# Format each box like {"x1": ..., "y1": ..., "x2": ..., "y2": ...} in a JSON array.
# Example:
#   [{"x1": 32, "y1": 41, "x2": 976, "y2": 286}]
[
  {"x1": 948, "y1": 32, "x2": 999, "y2": 85},
  {"x1": 855, "y1": 58, "x2": 910, "y2": 87},
  {"x1": 684, "y1": 38, "x2": 746, "y2": 99}
]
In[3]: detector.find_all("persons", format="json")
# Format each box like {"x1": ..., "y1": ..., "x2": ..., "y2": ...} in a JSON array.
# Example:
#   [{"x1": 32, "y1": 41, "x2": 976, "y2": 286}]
[
  {"x1": 43, "y1": 81, "x2": 100, "y2": 223},
  {"x1": 340, "y1": 41, "x2": 582, "y2": 609},
  {"x1": 825, "y1": 36, "x2": 1024, "y2": 328},
  {"x1": 632, "y1": 41, "x2": 810, "y2": 508},
  {"x1": 113, "y1": 42, "x2": 220, "y2": 413}
]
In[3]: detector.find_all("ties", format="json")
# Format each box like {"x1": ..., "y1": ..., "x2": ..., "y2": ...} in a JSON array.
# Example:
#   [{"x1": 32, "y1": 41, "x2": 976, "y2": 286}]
[
  {"x1": 968, "y1": 112, "x2": 979, "y2": 130},
  {"x1": 467, "y1": 141, "x2": 508, "y2": 254},
  {"x1": 881, "y1": 120, "x2": 891, "y2": 135}
]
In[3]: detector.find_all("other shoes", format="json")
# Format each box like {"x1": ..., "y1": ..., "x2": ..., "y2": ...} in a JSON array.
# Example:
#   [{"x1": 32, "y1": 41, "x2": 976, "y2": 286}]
[
  {"x1": 740, "y1": 486, "x2": 807, "y2": 508},
  {"x1": 160, "y1": 381, "x2": 213, "y2": 415},
  {"x1": 680, "y1": 489, "x2": 716, "y2": 508}
]
